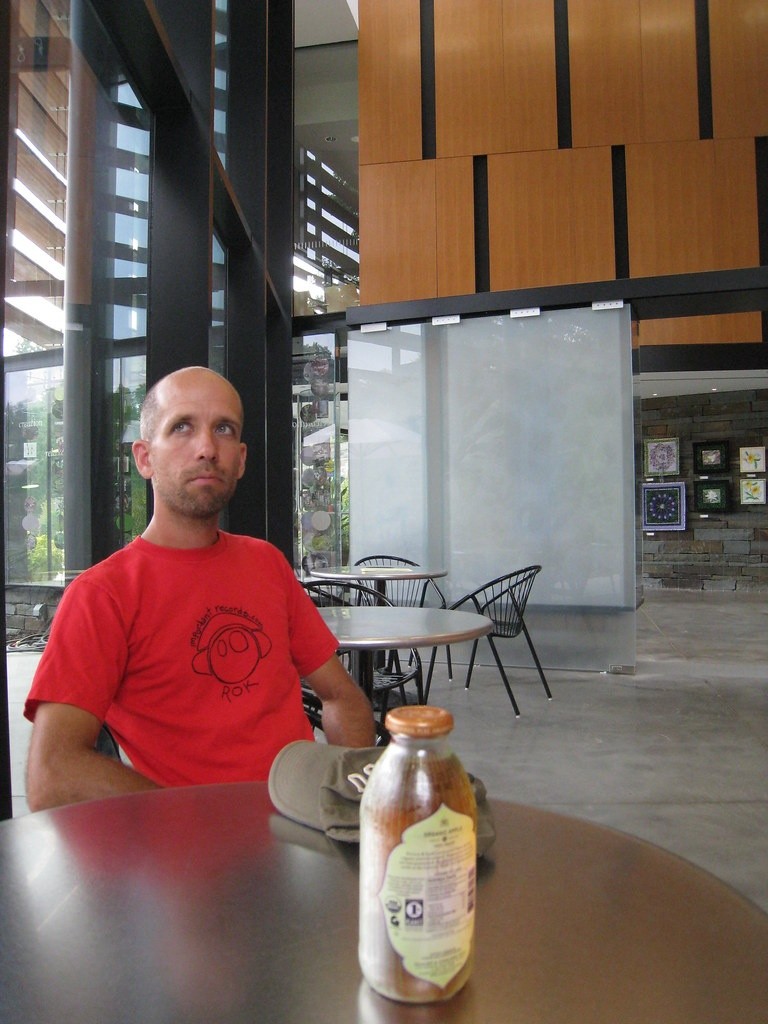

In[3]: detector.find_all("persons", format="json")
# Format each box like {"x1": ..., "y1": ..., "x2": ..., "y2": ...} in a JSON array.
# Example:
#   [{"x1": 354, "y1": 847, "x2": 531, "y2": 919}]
[{"x1": 21, "y1": 366, "x2": 375, "y2": 813}]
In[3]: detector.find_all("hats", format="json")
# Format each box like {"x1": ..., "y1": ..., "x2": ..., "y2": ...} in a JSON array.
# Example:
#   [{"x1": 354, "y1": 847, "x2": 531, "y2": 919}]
[{"x1": 268, "y1": 741, "x2": 497, "y2": 858}]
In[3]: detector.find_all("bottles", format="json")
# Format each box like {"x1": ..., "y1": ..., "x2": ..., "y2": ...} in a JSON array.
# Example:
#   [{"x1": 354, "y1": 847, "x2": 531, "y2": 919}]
[{"x1": 357, "y1": 705, "x2": 480, "y2": 1004}]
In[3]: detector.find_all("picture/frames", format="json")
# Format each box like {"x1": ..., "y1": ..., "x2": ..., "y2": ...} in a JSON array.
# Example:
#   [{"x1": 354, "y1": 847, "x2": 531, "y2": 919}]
[
  {"x1": 740, "y1": 445, "x2": 766, "y2": 473},
  {"x1": 693, "y1": 441, "x2": 732, "y2": 474},
  {"x1": 692, "y1": 478, "x2": 733, "y2": 511},
  {"x1": 642, "y1": 436, "x2": 682, "y2": 478},
  {"x1": 641, "y1": 482, "x2": 686, "y2": 531},
  {"x1": 739, "y1": 478, "x2": 766, "y2": 505}
]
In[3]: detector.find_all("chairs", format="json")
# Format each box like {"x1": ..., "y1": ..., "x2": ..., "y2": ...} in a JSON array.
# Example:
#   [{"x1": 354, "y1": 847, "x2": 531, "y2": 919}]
[{"x1": 81, "y1": 548, "x2": 556, "y2": 790}]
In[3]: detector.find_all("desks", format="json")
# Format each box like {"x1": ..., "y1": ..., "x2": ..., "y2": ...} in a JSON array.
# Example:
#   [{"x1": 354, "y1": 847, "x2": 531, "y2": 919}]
[
  {"x1": 0, "y1": 781, "x2": 767, "y2": 1023},
  {"x1": 282, "y1": 601, "x2": 498, "y2": 706},
  {"x1": 310, "y1": 566, "x2": 452, "y2": 711}
]
